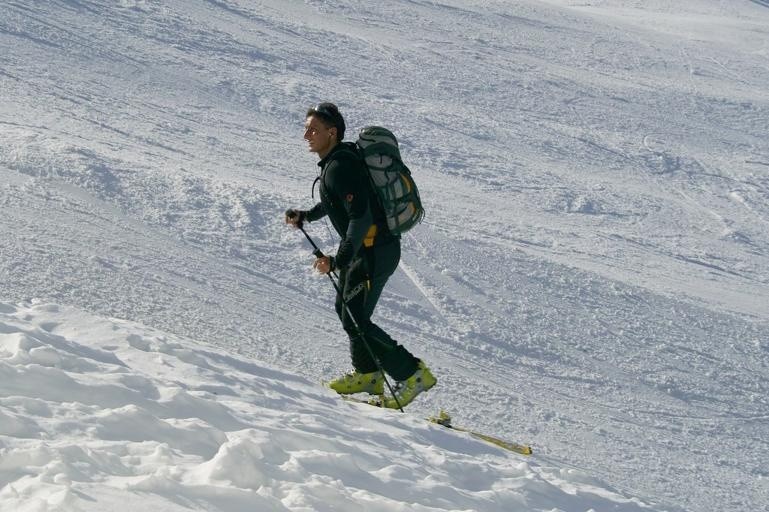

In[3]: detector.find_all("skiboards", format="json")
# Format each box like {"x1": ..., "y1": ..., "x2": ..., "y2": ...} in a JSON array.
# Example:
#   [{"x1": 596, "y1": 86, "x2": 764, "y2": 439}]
[{"x1": 322, "y1": 381, "x2": 531, "y2": 455}]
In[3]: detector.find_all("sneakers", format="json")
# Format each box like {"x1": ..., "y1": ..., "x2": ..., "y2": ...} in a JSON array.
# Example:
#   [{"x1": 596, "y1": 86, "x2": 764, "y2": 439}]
[
  {"x1": 329, "y1": 369, "x2": 385, "y2": 396},
  {"x1": 368, "y1": 361, "x2": 437, "y2": 410}
]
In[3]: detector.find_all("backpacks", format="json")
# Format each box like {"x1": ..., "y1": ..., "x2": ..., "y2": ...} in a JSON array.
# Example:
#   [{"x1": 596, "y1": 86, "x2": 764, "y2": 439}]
[{"x1": 320, "y1": 126, "x2": 424, "y2": 239}]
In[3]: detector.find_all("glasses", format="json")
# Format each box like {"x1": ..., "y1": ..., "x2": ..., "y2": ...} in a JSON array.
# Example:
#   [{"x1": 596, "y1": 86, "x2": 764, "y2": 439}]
[{"x1": 312, "y1": 103, "x2": 334, "y2": 120}]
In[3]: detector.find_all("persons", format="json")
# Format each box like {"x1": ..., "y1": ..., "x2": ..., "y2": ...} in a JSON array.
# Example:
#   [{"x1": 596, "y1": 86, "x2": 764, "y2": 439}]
[{"x1": 285, "y1": 103, "x2": 437, "y2": 410}]
